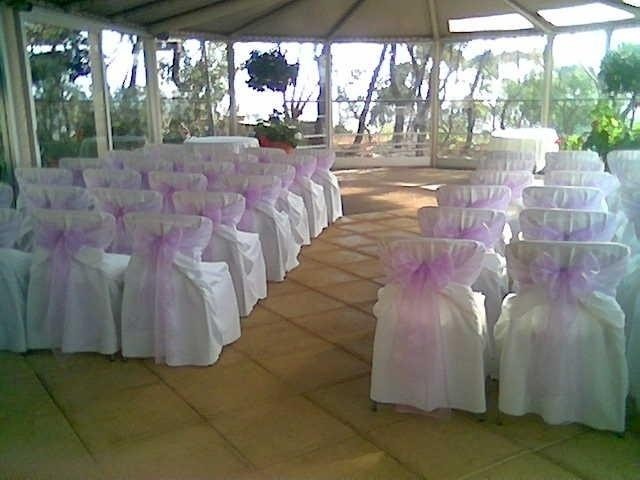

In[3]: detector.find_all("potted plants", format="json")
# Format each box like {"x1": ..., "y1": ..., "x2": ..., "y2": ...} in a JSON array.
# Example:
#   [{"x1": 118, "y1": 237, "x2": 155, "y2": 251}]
[{"x1": 254, "y1": 108, "x2": 302, "y2": 154}]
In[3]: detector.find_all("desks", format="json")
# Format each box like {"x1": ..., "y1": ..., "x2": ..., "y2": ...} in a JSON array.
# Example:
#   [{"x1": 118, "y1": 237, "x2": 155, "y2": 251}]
[
  {"x1": 492, "y1": 129, "x2": 559, "y2": 172},
  {"x1": 184, "y1": 136, "x2": 260, "y2": 152}
]
